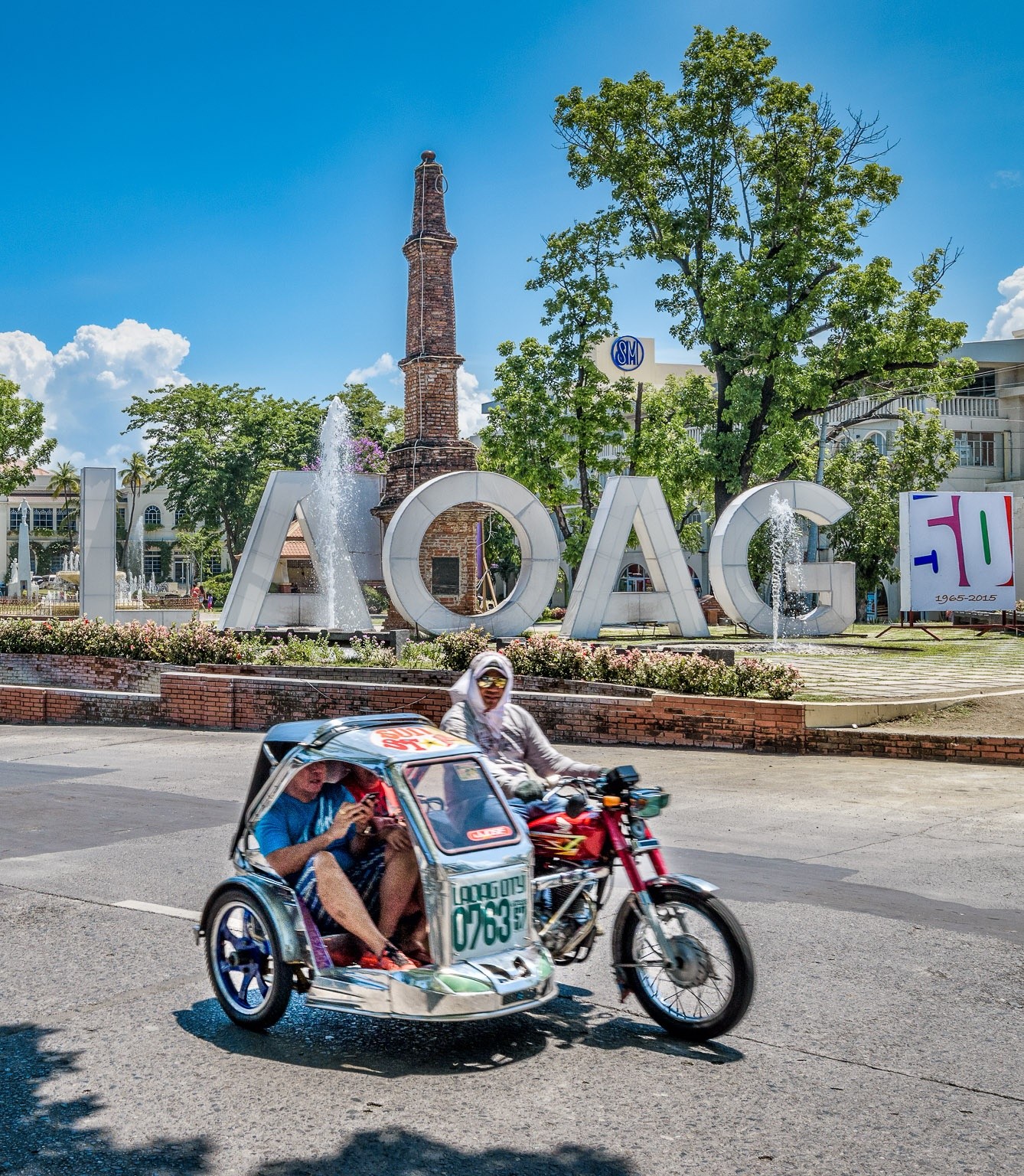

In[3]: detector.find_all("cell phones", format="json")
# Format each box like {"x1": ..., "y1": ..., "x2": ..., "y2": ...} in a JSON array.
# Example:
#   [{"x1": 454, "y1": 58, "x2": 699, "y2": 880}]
[{"x1": 359, "y1": 793, "x2": 380, "y2": 807}]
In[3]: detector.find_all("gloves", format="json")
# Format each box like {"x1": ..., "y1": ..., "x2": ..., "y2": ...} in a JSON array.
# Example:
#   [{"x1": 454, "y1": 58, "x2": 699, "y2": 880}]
[
  {"x1": 515, "y1": 780, "x2": 543, "y2": 803},
  {"x1": 595, "y1": 767, "x2": 613, "y2": 776}
]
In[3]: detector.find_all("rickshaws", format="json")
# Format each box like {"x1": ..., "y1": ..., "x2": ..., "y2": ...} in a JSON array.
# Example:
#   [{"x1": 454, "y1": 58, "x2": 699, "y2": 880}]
[{"x1": 193, "y1": 708, "x2": 756, "y2": 1047}]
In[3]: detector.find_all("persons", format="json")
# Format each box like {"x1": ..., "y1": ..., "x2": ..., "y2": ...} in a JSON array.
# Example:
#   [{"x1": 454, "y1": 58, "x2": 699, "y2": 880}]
[
  {"x1": 191, "y1": 584, "x2": 213, "y2": 612},
  {"x1": 256, "y1": 763, "x2": 431, "y2": 973},
  {"x1": 440, "y1": 652, "x2": 610, "y2": 937}
]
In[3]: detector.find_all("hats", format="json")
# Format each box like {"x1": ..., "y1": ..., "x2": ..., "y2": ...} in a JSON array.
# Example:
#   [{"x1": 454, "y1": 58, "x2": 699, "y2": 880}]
[{"x1": 476, "y1": 662, "x2": 506, "y2": 678}]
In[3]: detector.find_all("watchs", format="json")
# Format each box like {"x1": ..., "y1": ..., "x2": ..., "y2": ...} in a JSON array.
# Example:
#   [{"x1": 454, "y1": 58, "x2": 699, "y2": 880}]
[{"x1": 358, "y1": 823, "x2": 372, "y2": 834}]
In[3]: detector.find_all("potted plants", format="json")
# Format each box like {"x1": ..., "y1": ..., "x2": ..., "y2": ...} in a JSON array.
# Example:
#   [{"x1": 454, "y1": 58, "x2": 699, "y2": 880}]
[{"x1": 165, "y1": 577, "x2": 178, "y2": 592}]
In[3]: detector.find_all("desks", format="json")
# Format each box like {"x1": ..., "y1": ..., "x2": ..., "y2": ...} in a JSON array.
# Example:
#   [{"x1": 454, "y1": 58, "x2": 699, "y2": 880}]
[{"x1": 705, "y1": 608, "x2": 720, "y2": 627}]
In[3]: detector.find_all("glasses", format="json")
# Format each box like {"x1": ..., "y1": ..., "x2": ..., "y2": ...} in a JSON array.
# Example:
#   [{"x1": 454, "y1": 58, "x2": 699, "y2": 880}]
[{"x1": 476, "y1": 675, "x2": 507, "y2": 689}]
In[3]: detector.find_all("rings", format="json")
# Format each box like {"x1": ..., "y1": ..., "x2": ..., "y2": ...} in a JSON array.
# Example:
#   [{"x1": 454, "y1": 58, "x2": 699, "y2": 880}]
[{"x1": 339, "y1": 808, "x2": 346, "y2": 814}]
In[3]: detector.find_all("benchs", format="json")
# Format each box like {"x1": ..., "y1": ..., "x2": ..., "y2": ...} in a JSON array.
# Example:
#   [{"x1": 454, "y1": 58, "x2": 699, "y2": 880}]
[
  {"x1": 719, "y1": 617, "x2": 731, "y2": 626},
  {"x1": 245, "y1": 834, "x2": 357, "y2": 949}
]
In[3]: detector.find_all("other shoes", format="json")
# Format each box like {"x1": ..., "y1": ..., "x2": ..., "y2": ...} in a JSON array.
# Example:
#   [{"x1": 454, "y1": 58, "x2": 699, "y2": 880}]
[
  {"x1": 331, "y1": 947, "x2": 360, "y2": 967},
  {"x1": 360, "y1": 944, "x2": 418, "y2": 971}
]
